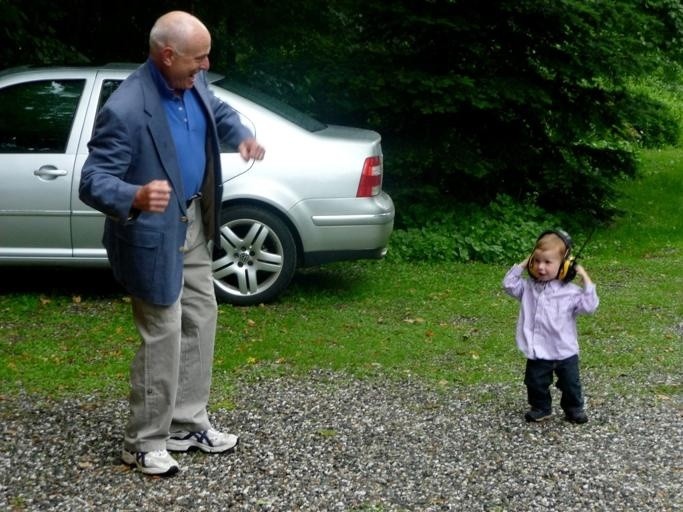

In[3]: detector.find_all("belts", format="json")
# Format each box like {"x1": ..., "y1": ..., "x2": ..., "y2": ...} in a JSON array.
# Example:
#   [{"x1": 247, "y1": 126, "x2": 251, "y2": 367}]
[{"x1": 183, "y1": 195, "x2": 200, "y2": 210}]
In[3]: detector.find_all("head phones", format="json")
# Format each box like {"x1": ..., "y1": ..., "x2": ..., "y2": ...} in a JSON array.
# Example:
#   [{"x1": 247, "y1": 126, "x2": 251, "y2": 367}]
[{"x1": 526, "y1": 228, "x2": 578, "y2": 285}]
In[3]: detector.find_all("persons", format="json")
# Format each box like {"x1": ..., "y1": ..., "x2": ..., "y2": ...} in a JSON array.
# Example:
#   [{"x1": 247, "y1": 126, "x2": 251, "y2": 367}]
[
  {"x1": 76, "y1": 8, "x2": 267, "y2": 478},
  {"x1": 500, "y1": 226, "x2": 600, "y2": 424}
]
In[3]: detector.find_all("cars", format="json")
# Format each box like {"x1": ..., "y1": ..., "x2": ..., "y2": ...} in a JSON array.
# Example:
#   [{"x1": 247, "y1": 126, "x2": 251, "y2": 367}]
[{"x1": 0, "y1": 57, "x2": 397, "y2": 306}]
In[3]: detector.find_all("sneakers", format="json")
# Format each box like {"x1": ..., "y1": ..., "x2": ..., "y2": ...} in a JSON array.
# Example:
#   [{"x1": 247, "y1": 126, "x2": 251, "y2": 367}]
[
  {"x1": 120, "y1": 444, "x2": 181, "y2": 478},
  {"x1": 165, "y1": 425, "x2": 240, "y2": 455},
  {"x1": 523, "y1": 406, "x2": 552, "y2": 423},
  {"x1": 564, "y1": 411, "x2": 588, "y2": 425}
]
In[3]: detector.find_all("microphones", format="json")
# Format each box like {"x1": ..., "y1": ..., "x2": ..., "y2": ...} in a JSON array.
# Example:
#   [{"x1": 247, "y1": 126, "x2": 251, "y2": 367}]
[{"x1": 574, "y1": 229, "x2": 594, "y2": 264}]
